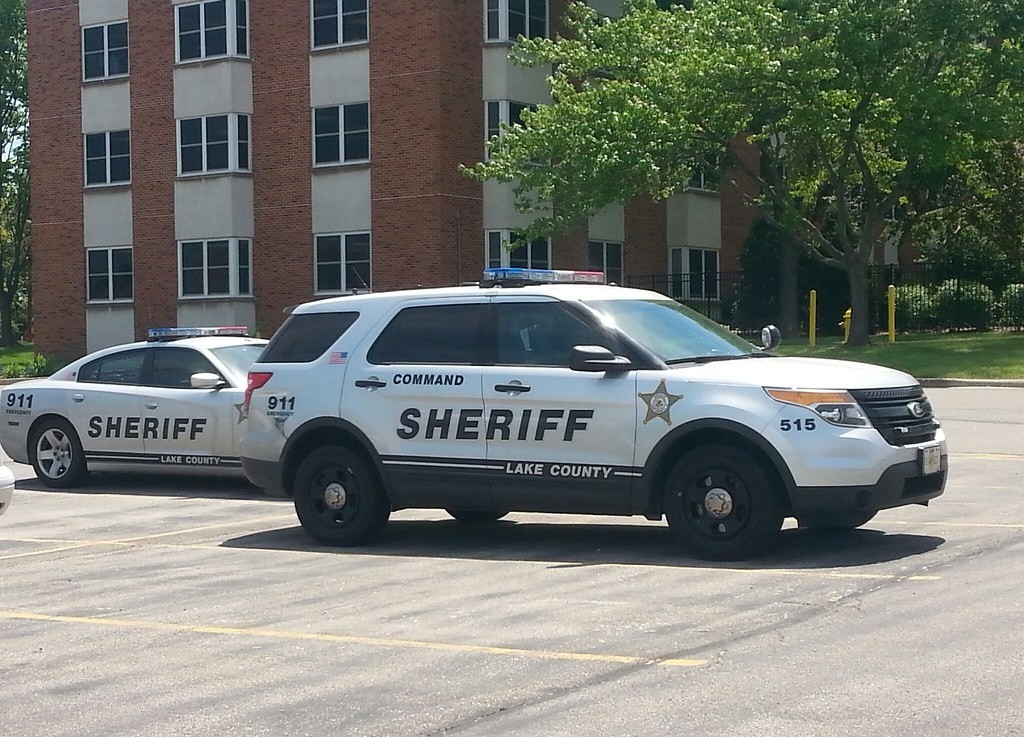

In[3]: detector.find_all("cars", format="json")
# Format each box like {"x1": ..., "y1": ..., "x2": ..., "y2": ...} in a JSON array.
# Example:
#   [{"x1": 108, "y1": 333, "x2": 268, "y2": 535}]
[{"x1": 1, "y1": 326, "x2": 273, "y2": 488}]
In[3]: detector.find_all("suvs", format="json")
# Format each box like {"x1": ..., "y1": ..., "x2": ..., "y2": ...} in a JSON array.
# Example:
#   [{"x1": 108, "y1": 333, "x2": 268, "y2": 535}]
[{"x1": 242, "y1": 267, "x2": 949, "y2": 562}]
